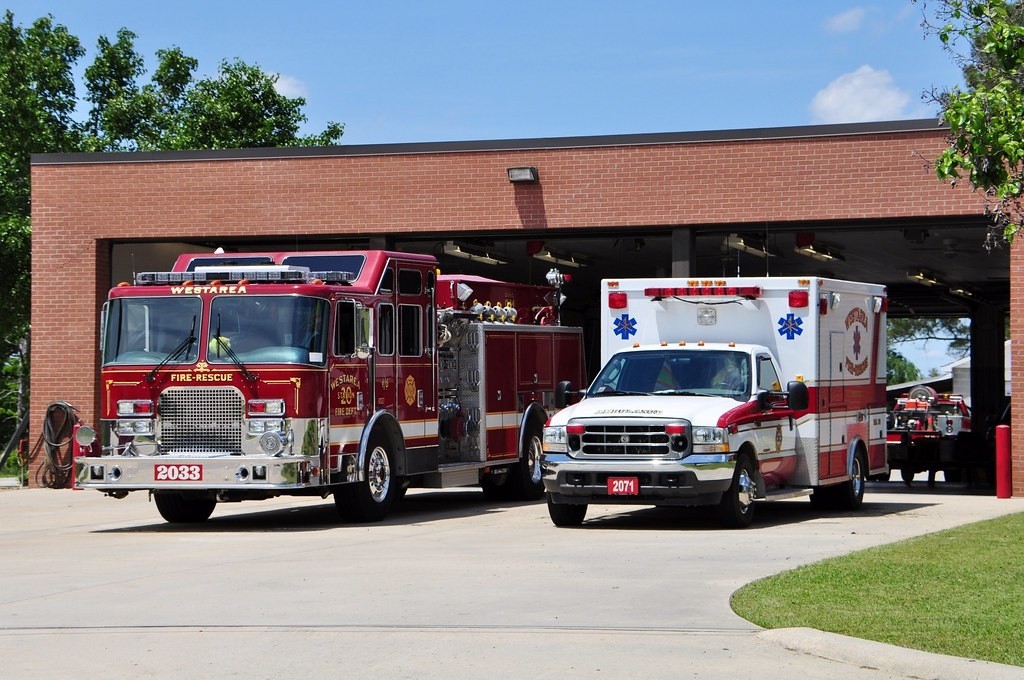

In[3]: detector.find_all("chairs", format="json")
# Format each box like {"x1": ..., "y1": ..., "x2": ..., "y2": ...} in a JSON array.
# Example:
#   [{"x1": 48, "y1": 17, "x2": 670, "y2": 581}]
[
  {"x1": 161, "y1": 313, "x2": 200, "y2": 354},
  {"x1": 708, "y1": 360, "x2": 743, "y2": 391},
  {"x1": 632, "y1": 365, "x2": 660, "y2": 392}
]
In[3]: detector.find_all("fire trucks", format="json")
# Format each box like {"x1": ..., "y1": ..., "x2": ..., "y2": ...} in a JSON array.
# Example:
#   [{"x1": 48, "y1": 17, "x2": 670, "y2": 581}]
[
  {"x1": 884, "y1": 385, "x2": 972, "y2": 486},
  {"x1": 75, "y1": 248, "x2": 588, "y2": 523}
]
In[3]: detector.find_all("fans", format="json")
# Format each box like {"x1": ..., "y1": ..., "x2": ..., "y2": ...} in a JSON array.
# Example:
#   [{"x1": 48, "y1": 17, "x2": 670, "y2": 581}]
[{"x1": 910, "y1": 239, "x2": 985, "y2": 259}]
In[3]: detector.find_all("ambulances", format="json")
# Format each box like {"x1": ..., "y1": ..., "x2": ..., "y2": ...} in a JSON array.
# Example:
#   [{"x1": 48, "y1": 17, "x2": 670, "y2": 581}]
[{"x1": 538, "y1": 277, "x2": 891, "y2": 528}]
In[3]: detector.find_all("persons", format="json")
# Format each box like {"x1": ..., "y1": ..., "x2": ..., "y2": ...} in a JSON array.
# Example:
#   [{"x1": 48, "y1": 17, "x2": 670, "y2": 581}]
[{"x1": 710, "y1": 358, "x2": 741, "y2": 390}]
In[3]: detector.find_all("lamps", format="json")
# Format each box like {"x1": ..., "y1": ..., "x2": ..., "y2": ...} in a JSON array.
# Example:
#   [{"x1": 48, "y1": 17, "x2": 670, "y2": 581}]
[
  {"x1": 905, "y1": 269, "x2": 943, "y2": 286},
  {"x1": 793, "y1": 243, "x2": 846, "y2": 263},
  {"x1": 532, "y1": 247, "x2": 594, "y2": 268},
  {"x1": 949, "y1": 285, "x2": 974, "y2": 299},
  {"x1": 507, "y1": 166, "x2": 540, "y2": 184},
  {"x1": 440, "y1": 241, "x2": 513, "y2": 266},
  {"x1": 722, "y1": 233, "x2": 777, "y2": 258}
]
